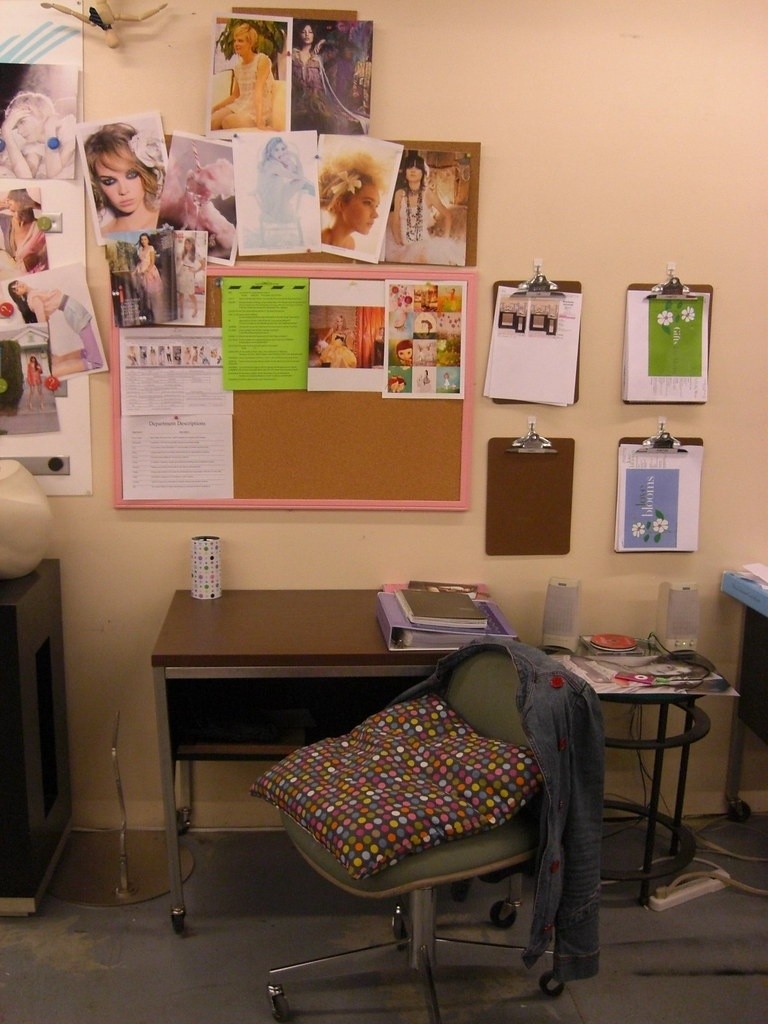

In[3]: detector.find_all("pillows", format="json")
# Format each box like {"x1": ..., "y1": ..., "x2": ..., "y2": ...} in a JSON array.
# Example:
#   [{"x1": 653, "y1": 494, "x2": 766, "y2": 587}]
[{"x1": 249, "y1": 690, "x2": 546, "y2": 882}]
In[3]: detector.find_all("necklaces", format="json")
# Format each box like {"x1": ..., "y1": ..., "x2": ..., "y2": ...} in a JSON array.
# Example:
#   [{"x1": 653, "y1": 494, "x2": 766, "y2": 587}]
[{"x1": 406, "y1": 190, "x2": 423, "y2": 243}]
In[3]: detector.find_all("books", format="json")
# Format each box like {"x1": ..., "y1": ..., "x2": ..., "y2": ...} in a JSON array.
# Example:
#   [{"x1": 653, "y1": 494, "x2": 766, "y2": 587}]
[{"x1": 394, "y1": 589, "x2": 488, "y2": 628}]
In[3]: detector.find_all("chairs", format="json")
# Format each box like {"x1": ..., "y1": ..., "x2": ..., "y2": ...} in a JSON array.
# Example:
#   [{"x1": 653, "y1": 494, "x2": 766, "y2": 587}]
[{"x1": 266, "y1": 632, "x2": 607, "y2": 1024}]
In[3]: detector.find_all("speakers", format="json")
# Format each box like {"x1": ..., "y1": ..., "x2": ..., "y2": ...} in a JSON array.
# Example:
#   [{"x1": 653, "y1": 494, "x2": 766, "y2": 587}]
[
  {"x1": 541, "y1": 577, "x2": 580, "y2": 653},
  {"x1": 656, "y1": 581, "x2": 699, "y2": 657}
]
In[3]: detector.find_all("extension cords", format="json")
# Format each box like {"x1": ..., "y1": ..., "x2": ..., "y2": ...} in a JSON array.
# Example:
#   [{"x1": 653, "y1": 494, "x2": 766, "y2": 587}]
[{"x1": 649, "y1": 869, "x2": 731, "y2": 912}]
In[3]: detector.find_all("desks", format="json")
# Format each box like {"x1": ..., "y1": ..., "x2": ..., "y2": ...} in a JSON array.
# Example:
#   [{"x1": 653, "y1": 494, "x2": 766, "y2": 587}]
[
  {"x1": 531, "y1": 641, "x2": 740, "y2": 905},
  {"x1": 150, "y1": 588, "x2": 522, "y2": 924},
  {"x1": 0, "y1": 558, "x2": 76, "y2": 918}
]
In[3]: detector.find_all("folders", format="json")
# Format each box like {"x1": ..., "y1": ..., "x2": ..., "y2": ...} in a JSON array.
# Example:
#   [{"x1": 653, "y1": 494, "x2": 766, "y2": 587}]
[{"x1": 376, "y1": 591, "x2": 518, "y2": 651}]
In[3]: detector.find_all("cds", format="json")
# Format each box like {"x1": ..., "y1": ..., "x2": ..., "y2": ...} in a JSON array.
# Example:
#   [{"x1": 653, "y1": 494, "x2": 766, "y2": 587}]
[{"x1": 591, "y1": 633, "x2": 637, "y2": 649}]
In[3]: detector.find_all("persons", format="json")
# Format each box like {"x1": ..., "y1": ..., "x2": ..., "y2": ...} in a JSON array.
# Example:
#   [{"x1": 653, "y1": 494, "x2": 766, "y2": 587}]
[
  {"x1": 318, "y1": 150, "x2": 386, "y2": 249},
  {"x1": 386, "y1": 155, "x2": 452, "y2": 263},
  {"x1": 6, "y1": 189, "x2": 48, "y2": 275},
  {"x1": 86, "y1": 122, "x2": 165, "y2": 233},
  {"x1": 7, "y1": 280, "x2": 101, "y2": 378},
  {"x1": 258, "y1": 137, "x2": 317, "y2": 220},
  {"x1": 211, "y1": 23, "x2": 273, "y2": 131},
  {"x1": 132, "y1": 232, "x2": 163, "y2": 318},
  {"x1": 293, "y1": 26, "x2": 341, "y2": 128},
  {"x1": 176, "y1": 237, "x2": 205, "y2": 317},
  {"x1": 2, "y1": 94, "x2": 75, "y2": 178},
  {"x1": 309, "y1": 315, "x2": 357, "y2": 368},
  {"x1": 127, "y1": 347, "x2": 221, "y2": 365},
  {"x1": 28, "y1": 356, "x2": 45, "y2": 411}
]
